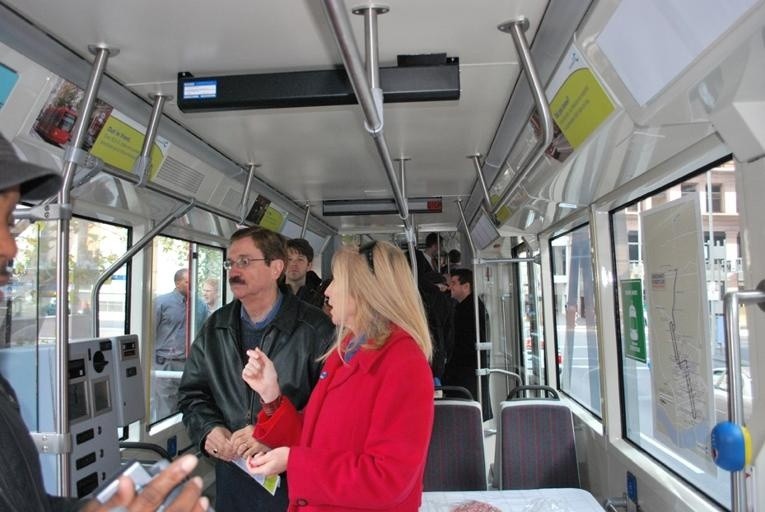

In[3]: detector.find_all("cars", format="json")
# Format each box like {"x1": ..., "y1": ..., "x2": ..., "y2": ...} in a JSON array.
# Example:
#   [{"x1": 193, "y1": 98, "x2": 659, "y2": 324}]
[{"x1": 523, "y1": 338, "x2": 563, "y2": 379}]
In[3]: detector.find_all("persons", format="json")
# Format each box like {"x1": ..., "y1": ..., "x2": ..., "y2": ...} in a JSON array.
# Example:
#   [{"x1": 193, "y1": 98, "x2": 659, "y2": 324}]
[
  {"x1": 242, "y1": 241, "x2": 434, "y2": 512},
  {"x1": 151, "y1": 269, "x2": 209, "y2": 422},
  {"x1": 203, "y1": 277, "x2": 219, "y2": 313},
  {"x1": 278, "y1": 239, "x2": 323, "y2": 309},
  {"x1": 0, "y1": 132, "x2": 209, "y2": 512},
  {"x1": 178, "y1": 229, "x2": 337, "y2": 512},
  {"x1": 404, "y1": 232, "x2": 493, "y2": 422}
]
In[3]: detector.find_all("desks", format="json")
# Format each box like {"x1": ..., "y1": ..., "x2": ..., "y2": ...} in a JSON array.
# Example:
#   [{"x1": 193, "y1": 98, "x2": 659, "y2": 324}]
[{"x1": 418, "y1": 488, "x2": 608, "y2": 512}]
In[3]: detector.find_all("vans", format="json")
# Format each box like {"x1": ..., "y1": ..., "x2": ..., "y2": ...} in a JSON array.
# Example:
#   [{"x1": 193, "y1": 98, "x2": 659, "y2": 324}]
[{"x1": 46, "y1": 297, "x2": 85, "y2": 316}]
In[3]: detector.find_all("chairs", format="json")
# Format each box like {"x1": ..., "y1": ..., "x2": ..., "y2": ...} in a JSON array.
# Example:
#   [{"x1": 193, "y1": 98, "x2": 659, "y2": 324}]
[
  {"x1": 426, "y1": 398, "x2": 487, "y2": 491},
  {"x1": 488, "y1": 399, "x2": 582, "y2": 491}
]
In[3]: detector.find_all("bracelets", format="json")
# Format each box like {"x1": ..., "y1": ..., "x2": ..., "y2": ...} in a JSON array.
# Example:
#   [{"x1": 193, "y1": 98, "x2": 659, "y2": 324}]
[{"x1": 259, "y1": 395, "x2": 282, "y2": 416}]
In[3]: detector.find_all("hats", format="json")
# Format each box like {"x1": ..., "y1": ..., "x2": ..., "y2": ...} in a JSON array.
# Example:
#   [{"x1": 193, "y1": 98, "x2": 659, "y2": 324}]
[{"x1": 0, "y1": 133, "x2": 64, "y2": 201}]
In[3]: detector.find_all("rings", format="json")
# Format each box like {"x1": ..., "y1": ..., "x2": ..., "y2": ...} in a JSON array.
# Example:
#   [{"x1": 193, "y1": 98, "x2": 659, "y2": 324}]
[
  {"x1": 213, "y1": 449, "x2": 218, "y2": 453},
  {"x1": 243, "y1": 443, "x2": 249, "y2": 449}
]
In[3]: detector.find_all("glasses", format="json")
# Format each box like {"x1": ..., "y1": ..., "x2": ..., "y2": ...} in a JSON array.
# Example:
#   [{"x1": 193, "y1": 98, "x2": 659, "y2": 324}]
[
  {"x1": 223, "y1": 257, "x2": 266, "y2": 268},
  {"x1": 359, "y1": 241, "x2": 379, "y2": 273}
]
